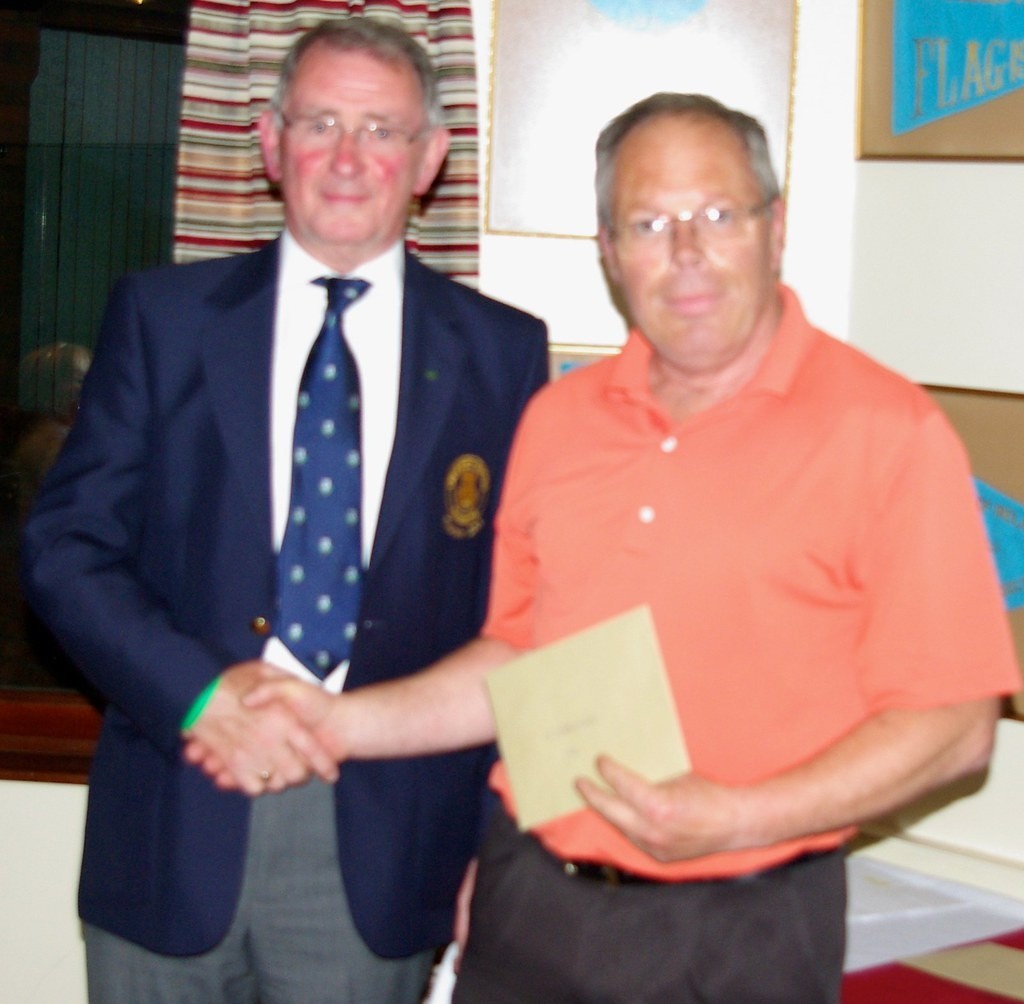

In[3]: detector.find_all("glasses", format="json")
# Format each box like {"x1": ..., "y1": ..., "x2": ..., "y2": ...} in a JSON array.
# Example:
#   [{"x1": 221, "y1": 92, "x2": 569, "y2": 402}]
[
  {"x1": 609, "y1": 198, "x2": 767, "y2": 250},
  {"x1": 284, "y1": 112, "x2": 418, "y2": 154}
]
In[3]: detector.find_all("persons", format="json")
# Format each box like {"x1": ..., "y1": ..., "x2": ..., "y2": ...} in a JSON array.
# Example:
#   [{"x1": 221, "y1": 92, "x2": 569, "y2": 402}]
[
  {"x1": 186, "y1": 93, "x2": 1020, "y2": 1003},
  {"x1": 15, "y1": 17, "x2": 551, "y2": 1004}
]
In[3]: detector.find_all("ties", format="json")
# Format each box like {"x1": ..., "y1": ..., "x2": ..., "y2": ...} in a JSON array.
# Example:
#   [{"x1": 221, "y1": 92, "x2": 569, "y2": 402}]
[{"x1": 275, "y1": 276, "x2": 372, "y2": 682}]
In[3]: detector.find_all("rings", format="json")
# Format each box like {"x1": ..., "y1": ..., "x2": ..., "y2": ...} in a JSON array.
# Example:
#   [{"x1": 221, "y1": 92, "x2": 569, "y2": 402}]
[{"x1": 257, "y1": 762, "x2": 276, "y2": 782}]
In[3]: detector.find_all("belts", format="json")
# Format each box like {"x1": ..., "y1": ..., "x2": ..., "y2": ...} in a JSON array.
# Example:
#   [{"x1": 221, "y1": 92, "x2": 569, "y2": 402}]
[{"x1": 522, "y1": 824, "x2": 655, "y2": 886}]
